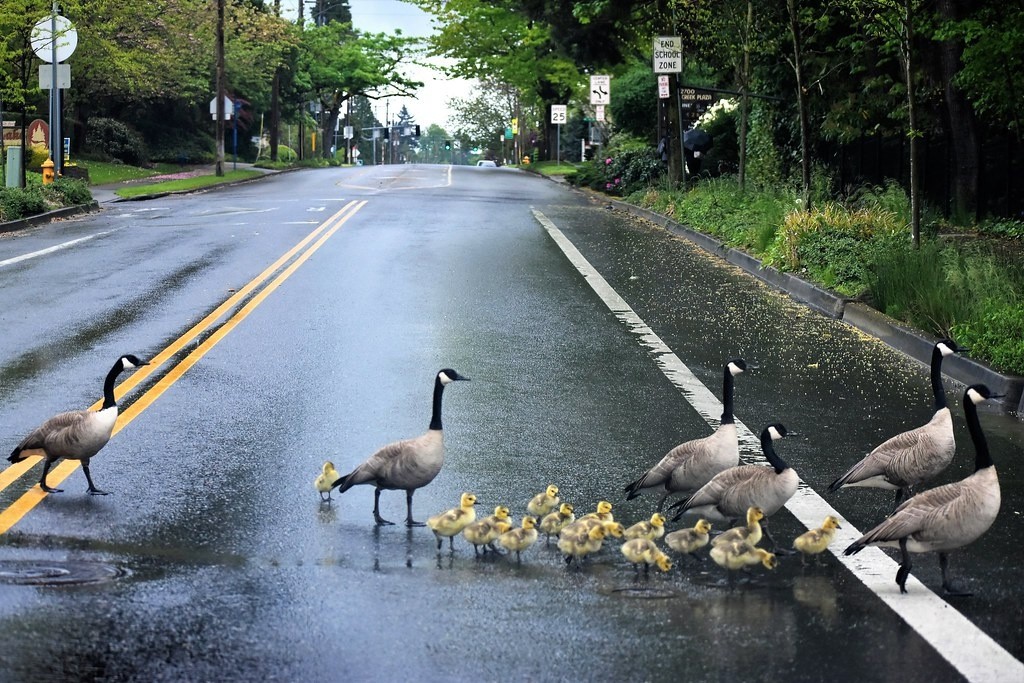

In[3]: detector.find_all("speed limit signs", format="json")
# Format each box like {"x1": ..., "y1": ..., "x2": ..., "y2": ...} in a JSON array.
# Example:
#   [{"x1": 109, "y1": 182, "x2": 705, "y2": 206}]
[{"x1": 551, "y1": 104, "x2": 567, "y2": 124}]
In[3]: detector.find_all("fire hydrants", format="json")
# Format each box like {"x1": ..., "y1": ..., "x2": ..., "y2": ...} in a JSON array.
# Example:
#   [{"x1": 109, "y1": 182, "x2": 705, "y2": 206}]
[
  {"x1": 522, "y1": 156, "x2": 530, "y2": 165},
  {"x1": 40, "y1": 157, "x2": 61, "y2": 184}
]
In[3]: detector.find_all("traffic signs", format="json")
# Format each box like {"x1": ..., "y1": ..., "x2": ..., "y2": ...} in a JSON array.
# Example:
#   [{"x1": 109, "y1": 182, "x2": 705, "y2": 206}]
[
  {"x1": 652, "y1": 36, "x2": 683, "y2": 76},
  {"x1": 596, "y1": 105, "x2": 604, "y2": 122},
  {"x1": 657, "y1": 75, "x2": 670, "y2": 99},
  {"x1": 590, "y1": 75, "x2": 610, "y2": 104}
]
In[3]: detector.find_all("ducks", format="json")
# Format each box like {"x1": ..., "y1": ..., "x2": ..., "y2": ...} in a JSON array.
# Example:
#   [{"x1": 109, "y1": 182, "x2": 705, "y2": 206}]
[
  {"x1": 664, "y1": 518, "x2": 712, "y2": 566},
  {"x1": 462, "y1": 506, "x2": 539, "y2": 570},
  {"x1": 556, "y1": 501, "x2": 625, "y2": 570},
  {"x1": 622, "y1": 357, "x2": 759, "y2": 515},
  {"x1": 823, "y1": 339, "x2": 974, "y2": 514},
  {"x1": 425, "y1": 492, "x2": 482, "y2": 553},
  {"x1": 708, "y1": 540, "x2": 778, "y2": 593},
  {"x1": 843, "y1": 382, "x2": 1007, "y2": 597},
  {"x1": 331, "y1": 367, "x2": 472, "y2": 526},
  {"x1": 315, "y1": 461, "x2": 338, "y2": 502},
  {"x1": 711, "y1": 507, "x2": 769, "y2": 550},
  {"x1": 527, "y1": 485, "x2": 560, "y2": 523},
  {"x1": 537, "y1": 503, "x2": 575, "y2": 547},
  {"x1": 619, "y1": 537, "x2": 672, "y2": 582},
  {"x1": 791, "y1": 516, "x2": 843, "y2": 567},
  {"x1": 666, "y1": 424, "x2": 800, "y2": 556},
  {"x1": 7, "y1": 353, "x2": 150, "y2": 495},
  {"x1": 622, "y1": 513, "x2": 669, "y2": 542}
]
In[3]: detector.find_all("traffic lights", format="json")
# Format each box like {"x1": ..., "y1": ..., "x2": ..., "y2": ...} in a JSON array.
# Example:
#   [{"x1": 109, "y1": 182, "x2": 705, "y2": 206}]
[
  {"x1": 445, "y1": 140, "x2": 451, "y2": 150},
  {"x1": 472, "y1": 143, "x2": 478, "y2": 152}
]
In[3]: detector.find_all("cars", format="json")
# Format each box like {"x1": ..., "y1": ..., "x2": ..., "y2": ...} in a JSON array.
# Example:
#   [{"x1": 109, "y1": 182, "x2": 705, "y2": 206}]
[{"x1": 476, "y1": 160, "x2": 497, "y2": 167}]
